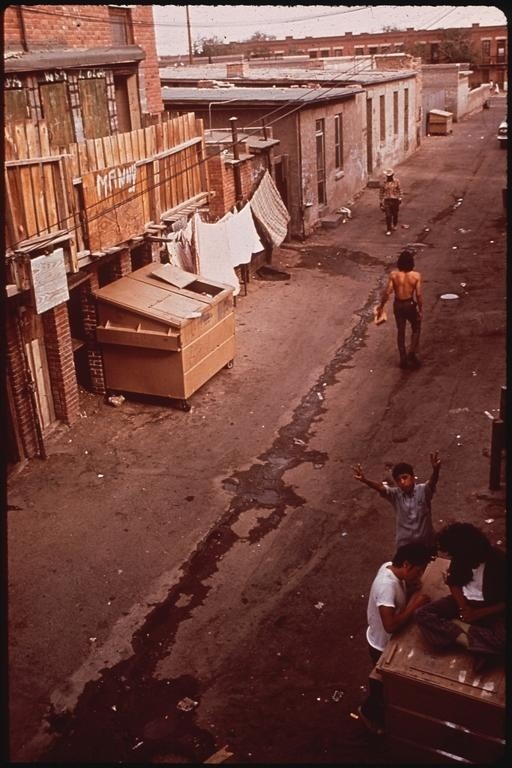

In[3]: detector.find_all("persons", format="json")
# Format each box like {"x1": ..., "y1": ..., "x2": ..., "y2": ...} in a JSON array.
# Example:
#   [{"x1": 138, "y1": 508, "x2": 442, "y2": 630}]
[
  {"x1": 407, "y1": 518, "x2": 510, "y2": 672},
  {"x1": 348, "y1": 446, "x2": 445, "y2": 543},
  {"x1": 347, "y1": 541, "x2": 431, "y2": 738},
  {"x1": 377, "y1": 167, "x2": 404, "y2": 234},
  {"x1": 372, "y1": 249, "x2": 423, "y2": 369}
]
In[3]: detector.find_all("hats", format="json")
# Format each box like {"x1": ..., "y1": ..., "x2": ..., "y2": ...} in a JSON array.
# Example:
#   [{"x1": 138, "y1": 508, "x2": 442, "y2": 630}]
[{"x1": 382, "y1": 167, "x2": 396, "y2": 178}]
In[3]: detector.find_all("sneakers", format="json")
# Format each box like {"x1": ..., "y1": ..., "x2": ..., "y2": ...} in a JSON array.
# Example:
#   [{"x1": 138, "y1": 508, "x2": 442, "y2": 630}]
[{"x1": 356, "y1": 705, "x2": 386, "y2": 736}]
